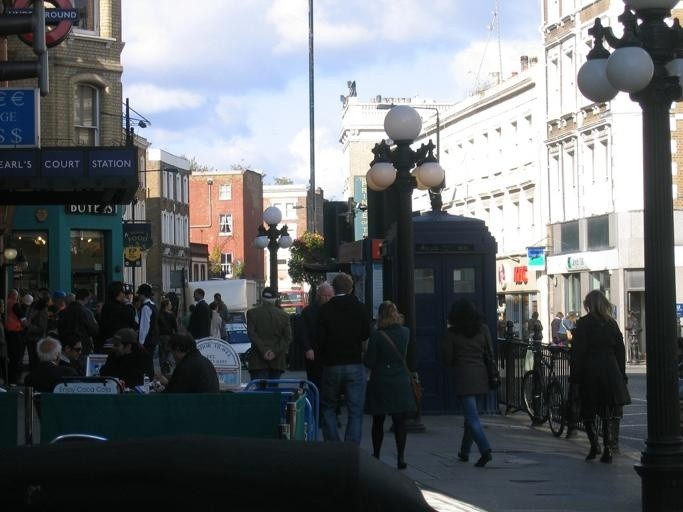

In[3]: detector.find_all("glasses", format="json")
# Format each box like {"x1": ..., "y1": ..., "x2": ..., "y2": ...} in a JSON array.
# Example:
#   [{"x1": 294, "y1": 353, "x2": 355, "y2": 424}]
[{"x1": 72, "y1": 347, "x2": 82, "y2": 352}]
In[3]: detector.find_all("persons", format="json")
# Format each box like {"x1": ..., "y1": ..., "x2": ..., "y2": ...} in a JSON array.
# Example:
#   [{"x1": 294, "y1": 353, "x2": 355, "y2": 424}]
[
  {"x1": 244, "y1": 286, "x2": 292, "y2": 388},
  {"x1": 438, "y1": 297, "x2": 501, "y2": 467},
  {"x1": 296, "y1": 281, "x2": 342, "y2": 429},
  {"x1": 550, "y1": 312, "x2": 563, "y2": 341},
  {"x1": 558, "y1": 311, "x2": 576, "y2": 339},
  {"x1": 525, "y1": 311, "x2": 543, "y2": 341},
  {"x1": 0, "y1": 280, "x2": 228, "y2": 394},
  {"x1": 311, "y1": 273, "x2": 369, "y2": 445},
  {"x1": 361, "y1": 300, "x2": 417, "y2": 469},
  {"x1": 568, "y1": 288, "x2": 631, "y2": 465}
]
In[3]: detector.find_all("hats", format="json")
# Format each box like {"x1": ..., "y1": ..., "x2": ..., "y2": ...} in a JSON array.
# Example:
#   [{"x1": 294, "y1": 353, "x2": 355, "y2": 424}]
[
  {"x1": 135, "y1": 284, "x2": 156, "y2": 296},
  {"x1": 113, "y1": 327, "x2": 138, "y2": 344},
  {"x1": 22, "y1": 294, "x2": 34, "y2": 306},
  {"x1": 261, "y1": 286, "x2": 279, "y2": 299}
]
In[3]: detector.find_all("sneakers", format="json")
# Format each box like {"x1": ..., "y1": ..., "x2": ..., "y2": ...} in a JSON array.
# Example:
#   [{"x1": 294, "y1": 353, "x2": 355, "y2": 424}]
[
  {"x1": 474, "y1": 454, "x2": 493, "y2": 467},
  {"x1": 457, "y1": 449, "x2": 470, "y2": 462}
]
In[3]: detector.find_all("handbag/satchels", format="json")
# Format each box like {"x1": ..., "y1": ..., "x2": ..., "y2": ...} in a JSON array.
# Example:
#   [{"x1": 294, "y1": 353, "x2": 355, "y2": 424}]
[
  {"x1": 488, "y1": 358, "x2": 505, "y2": 390},
  {"x1": 411, "y1": 378, "x2": 425, "y2": 400}
]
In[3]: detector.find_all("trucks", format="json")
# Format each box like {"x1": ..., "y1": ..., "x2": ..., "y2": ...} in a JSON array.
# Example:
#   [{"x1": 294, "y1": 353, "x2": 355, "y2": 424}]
[{"x1": 278, "y1": 286, "x2": 307, "y2": 314}]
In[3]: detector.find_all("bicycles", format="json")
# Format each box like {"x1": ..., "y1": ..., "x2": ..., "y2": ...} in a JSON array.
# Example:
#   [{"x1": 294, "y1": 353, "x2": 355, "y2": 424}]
[{"x1": 516, "y1": 338, "x2": 569, "y2": 438}]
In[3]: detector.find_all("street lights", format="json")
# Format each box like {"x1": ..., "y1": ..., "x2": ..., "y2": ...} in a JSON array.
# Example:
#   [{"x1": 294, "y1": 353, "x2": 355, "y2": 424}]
[
  {"x1": 16, "y1": 248, "x2": 30, "y2": 270},
  {"x1": 1, "y1": 242, "x2": 18, "y2": 321},
  {"x1": 253, "y1": 206, "x2": 292, "y2": 310},
  {"x1": 575, "y1": 0, "x2": 682, "y2": 510},
  {"x1": 366, "y1": 107, "x2": 445, "y2": 435}
]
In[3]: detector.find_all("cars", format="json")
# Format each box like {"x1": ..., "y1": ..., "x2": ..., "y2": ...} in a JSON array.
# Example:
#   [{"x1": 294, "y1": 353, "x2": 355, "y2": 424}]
[
  {"x1": 223, "y1": 322, "x2": 255, "y2": 371},
  {"x1": 217, "y1": 306, "x2": 250, "y2": 328}
]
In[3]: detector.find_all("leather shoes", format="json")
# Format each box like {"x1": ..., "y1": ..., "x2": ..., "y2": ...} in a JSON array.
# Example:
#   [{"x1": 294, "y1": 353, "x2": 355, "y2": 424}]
[{"x1": 397, "y1": 462, "x2": 408, "y2": 469}]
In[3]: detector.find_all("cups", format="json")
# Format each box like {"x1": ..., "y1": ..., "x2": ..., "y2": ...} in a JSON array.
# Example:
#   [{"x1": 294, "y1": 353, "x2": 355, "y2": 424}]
[
  {"x1": 286, "y1": 402, "x2": 297, "y2": 440},
  {"x1": 278, "y1": 424, "x2": 289, "y2": 440}
]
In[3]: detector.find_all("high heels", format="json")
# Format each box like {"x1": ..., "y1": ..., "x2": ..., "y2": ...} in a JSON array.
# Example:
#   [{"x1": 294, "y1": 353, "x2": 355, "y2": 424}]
[
  {"x1": 599, "y1": 446, "x2": 614, "y2": 464},
  {"x1": 586, "y1": 444, "x2": 601, "y2": 460}
]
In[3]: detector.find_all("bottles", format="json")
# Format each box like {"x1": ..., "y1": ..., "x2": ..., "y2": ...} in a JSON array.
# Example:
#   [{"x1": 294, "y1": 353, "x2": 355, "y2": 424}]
[{"x1": 143, "y1": 374, "x2": 149, "y2": 393}]
